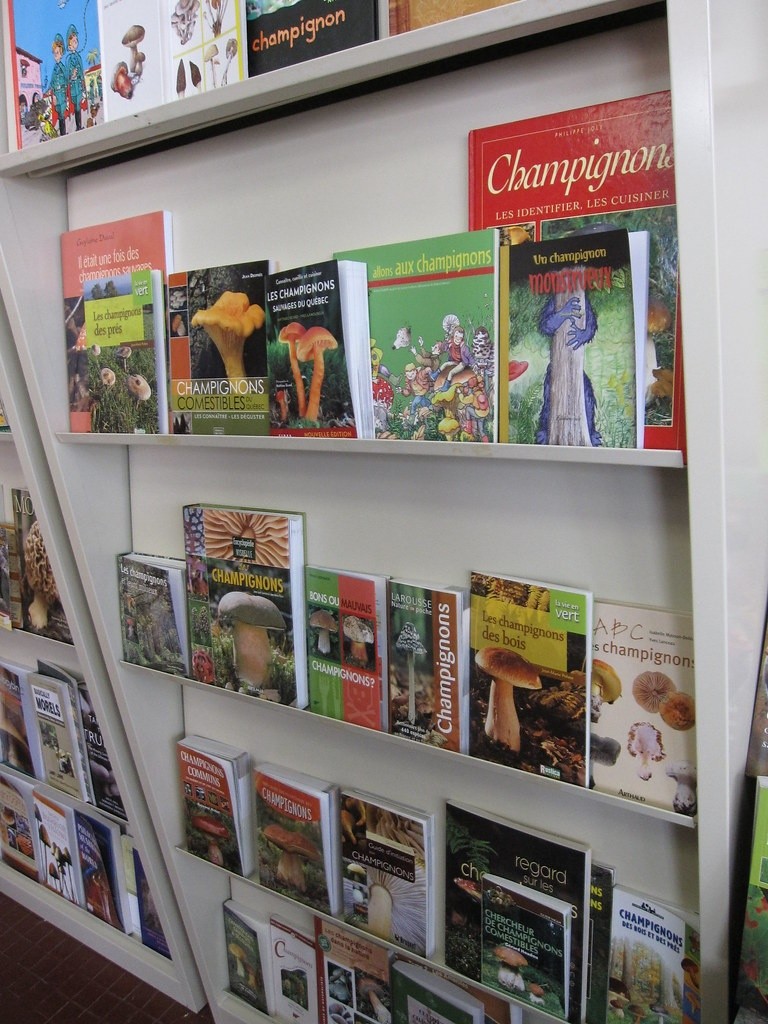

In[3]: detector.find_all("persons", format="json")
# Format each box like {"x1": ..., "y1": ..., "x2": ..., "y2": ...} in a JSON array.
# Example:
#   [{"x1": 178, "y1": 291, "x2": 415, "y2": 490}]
[{"x1": 61, "y1": 88, "x2": 687, "y2": 464}]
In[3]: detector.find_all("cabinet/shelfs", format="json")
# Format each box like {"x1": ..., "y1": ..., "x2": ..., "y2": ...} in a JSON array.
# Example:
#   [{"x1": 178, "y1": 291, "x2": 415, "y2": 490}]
[{"x1": 0, "y1": 0, "x2": 768, "y2": 1024}]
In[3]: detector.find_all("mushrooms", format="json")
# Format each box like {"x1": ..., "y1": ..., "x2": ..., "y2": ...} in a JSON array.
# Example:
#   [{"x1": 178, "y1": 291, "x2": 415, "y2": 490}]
[{"x1": 0, "y1": 0, "x2": 701, "y2": 1024}]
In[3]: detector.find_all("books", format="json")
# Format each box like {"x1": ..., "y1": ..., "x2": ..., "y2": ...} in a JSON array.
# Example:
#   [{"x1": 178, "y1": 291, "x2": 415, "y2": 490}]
[
  {"x1": 735, "y1": 774, "x2": 768, "y2": 1022},
  {"x1": 0, "y1": 399, "x2": 10, "y2": 432},
  {"x1": 2, "y1": 0, "x2": 521, "y2": 150},
  {"x1": 114, "y1": 504, "x2": 697, "y2": 820},
  {"x1": 0, "y1": 486, "x2": 172, "y2": 960},
  {"x1": 178, "y1": 734, "x2": 700, "y2": 1024}
]
